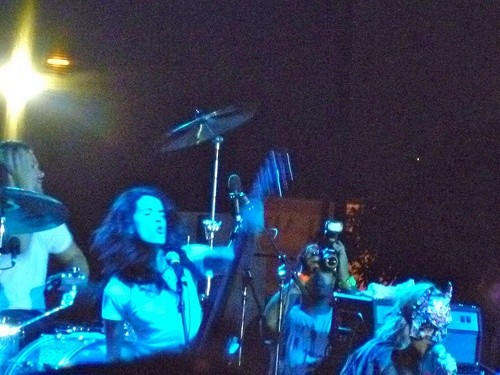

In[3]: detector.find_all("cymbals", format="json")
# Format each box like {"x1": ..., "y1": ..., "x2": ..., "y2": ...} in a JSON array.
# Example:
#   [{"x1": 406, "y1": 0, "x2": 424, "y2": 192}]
[
  {"x1": 161, "y1": 102, "x2": 259, "y2": 152},
  {"x1": 0, "y1": 184, "x2": 72, "y2": 236}
]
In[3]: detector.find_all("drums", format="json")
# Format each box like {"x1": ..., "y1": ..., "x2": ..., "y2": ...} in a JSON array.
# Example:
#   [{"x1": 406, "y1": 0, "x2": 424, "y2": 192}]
[{"x1": 5, "y1": 331, "x2": 137, "y2": 375}]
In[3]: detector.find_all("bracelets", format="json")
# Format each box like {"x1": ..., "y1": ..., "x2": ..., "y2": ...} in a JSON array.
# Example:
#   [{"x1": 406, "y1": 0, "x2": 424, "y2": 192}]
[{"x1": 339, "y1": 275, "x2": 357, "y2": 292}]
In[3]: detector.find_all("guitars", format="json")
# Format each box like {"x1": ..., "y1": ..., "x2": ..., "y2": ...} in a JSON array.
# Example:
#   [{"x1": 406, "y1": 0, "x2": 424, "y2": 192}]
[{"x1": 192, "y1": 148, "x2": 295, "y2": 346}]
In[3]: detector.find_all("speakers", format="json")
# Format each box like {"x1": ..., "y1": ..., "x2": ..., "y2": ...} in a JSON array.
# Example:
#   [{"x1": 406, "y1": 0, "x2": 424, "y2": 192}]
[{"x1": 373, "y1": 299, "x2": 483, "y2": 363}]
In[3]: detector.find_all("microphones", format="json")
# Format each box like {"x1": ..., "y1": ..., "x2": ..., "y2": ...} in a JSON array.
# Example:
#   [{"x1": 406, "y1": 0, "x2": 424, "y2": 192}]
[
  {"x1": 165, "y1": 252, "x2": 183, "y2": 277},
  {"x1": 226, "y1": 174, "x2": 241, "y2": 221},
  {"x1": 431, "y1": 344, "x2": 457, "y2": 375},
  {"x1": 7, "y1": 237, "x2": 21, "y2": 265}
]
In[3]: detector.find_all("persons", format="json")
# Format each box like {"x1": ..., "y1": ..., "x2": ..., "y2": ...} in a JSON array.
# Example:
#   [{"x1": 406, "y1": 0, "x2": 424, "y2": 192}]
[
  {"x1": 0, "y1": 141, "x2": 89, "y2": 313},
  {"x1": 339, "y1": 282, "x2": 457, "y2": 374},
  {"x1": 260, "y1": 239, "x2": 359, "y2": 375},
  {"x1": 89, "y1": 187, "x2": 247, "y2": 363}
]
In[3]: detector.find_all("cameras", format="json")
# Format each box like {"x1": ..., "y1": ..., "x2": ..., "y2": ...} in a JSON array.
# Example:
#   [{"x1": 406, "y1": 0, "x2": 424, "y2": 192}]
[{"x1": 311, "y1": 220, "x2": 343, "y2": 272}]
